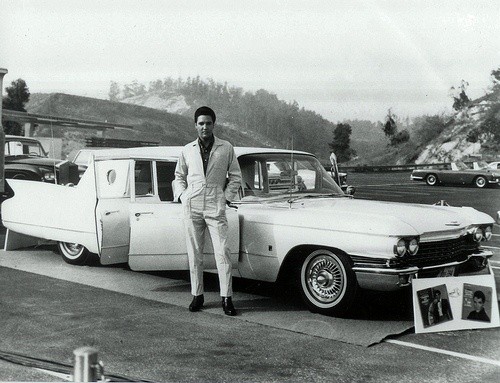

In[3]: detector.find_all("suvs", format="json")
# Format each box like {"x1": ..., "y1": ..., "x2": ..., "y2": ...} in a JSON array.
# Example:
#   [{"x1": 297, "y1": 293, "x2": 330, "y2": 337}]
[{"x1": 4, "y1": 130, "x2": 78, "y2": 195}]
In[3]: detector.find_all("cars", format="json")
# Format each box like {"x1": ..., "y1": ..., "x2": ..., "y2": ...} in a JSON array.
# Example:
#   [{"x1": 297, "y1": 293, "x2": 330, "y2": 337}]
[
  {"x1": 68, "y1": 147, "x2": 105, "y2": 177},
  {"x1": 464, "y1": 160, "x2": 495, "y2": 170},
  {"x1": 1, "y1": 147, "x2": 496, "y2": 317}
]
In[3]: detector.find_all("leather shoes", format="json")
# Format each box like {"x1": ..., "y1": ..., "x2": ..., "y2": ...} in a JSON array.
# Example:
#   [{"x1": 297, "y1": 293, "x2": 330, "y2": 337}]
[
  {"x1": 221, "y1": 296, "x2": 237, "y2": 316},
  {"x1": 189, "y1": 294, "x2": 204, "y2": 312}
]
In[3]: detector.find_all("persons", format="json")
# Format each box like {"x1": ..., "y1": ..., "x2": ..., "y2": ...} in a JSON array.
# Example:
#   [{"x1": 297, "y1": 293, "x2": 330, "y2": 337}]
[
  {"x1": 468, "y1": 292, "x2": 490, "y2": 323},
  {"x1": 429, "y1": 290, "x2": 453, "y2": 325},
  {"x1": 174, "y1": 106, "x2": 242, "y2": 316}
]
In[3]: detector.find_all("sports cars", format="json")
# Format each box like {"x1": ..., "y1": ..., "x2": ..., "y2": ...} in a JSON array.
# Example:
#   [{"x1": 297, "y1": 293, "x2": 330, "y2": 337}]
[{"x1": 410, "y1": 161, "x2": 500, "y2": 187}]
